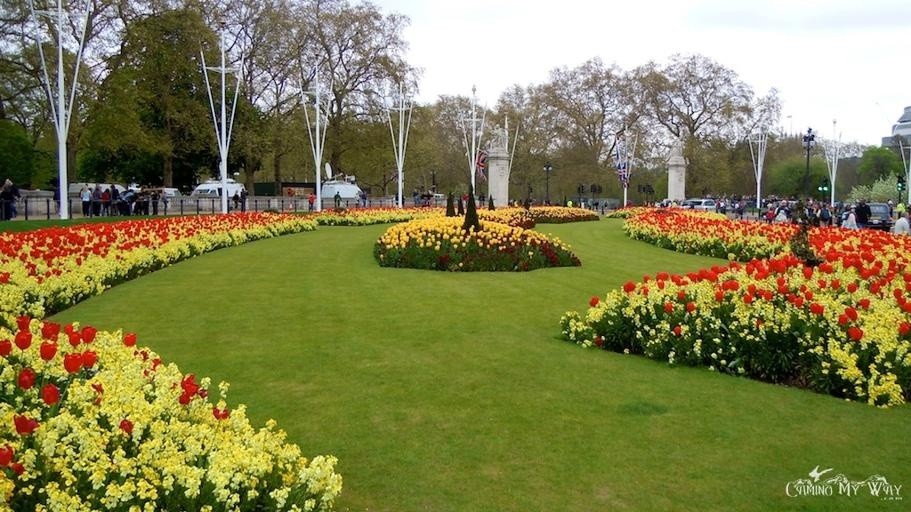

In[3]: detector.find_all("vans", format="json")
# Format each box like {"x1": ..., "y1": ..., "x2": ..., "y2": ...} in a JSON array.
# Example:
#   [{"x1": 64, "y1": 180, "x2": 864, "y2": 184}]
[
  {"x1": 679, "y1": 199, "x2": 717, "y2": 211},
  {"x1": 68, "y1": 181, "x2": 128, "y2": 214},
  {"x1": 148, "y1": 187, "x2": 182, "y2": 211},
  {"x1": 190, "y1": 178, "x2": 248, "y2": 212}
]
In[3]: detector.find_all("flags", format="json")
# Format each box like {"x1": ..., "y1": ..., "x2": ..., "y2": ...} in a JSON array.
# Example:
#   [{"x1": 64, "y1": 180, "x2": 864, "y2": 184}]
[
  {"x1": 474, "y1": 148, "x2": 489, "y2": 181},
  {"x1": 614, "y1": 140, "x2": 633, "y2": 190}
]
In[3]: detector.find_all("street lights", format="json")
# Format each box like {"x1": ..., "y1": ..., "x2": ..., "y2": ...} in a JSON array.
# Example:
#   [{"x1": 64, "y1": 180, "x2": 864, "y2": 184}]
[
  {"x1": 429, "y1": 168, "x2": 437, "y2": 193},
  {"x1": 800, "y1": 127, "x2": 817, "y2": 198},
  {"x1": 543, "y1": 160, "x2": 553, "y2": 203}
]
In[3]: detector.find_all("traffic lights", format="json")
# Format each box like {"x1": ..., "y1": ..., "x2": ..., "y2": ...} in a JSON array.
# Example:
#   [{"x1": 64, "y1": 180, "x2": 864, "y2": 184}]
[
  {"x1": 579, "y1": 183, "x2": 584, "y2": 194},
  {"x1": 896, "y1": 174, "x2": 903, "y2": 192},
  {"x1": 817, "y1": 178, "x2": 829, "y2": 193}
]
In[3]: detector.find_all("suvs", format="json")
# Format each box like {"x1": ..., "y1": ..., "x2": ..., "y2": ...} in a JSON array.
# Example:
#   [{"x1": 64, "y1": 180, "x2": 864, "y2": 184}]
[{"x1": 863, "y1": 202, "x2": 894, "y2": 232}]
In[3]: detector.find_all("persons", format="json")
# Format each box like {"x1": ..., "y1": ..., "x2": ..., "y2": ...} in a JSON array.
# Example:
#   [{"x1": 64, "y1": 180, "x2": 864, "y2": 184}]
[
  {"x1": 232, "y1": 189, "x2": 243, "y2": 209},
  {"x1": 238, "y1": 187, "x2": 251, "y2": 213},
  {"x1": 78, "y1": 183, "x2": 169, "y2": 215},
  {"x1": 332, "y1": 185, "x2": 610, "y2": 218},
  {"x1": 715, "y1": 191, "x2": 911, "y2": 235},
  {"x1": 646, "y1": 196, "x2": 696, "y2": 209},
  {"x1": 52, "y1": 182, "x2": 61, "y2": 217},
  {"x1": 307, "y1": 192, "x2": 317, "y2": 212},
  {"x1": 0, "y1": 177, "x2": 22, "y2": 221}
]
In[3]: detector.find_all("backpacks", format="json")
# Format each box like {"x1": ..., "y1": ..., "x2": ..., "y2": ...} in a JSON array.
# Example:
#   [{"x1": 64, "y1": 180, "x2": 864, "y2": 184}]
[
  {"x1": 842, "y1": 212, "x2": 849, "y2": 220},
  {"x1": 821, "y1": 208, "x2": 828, "y2": 221}
]
